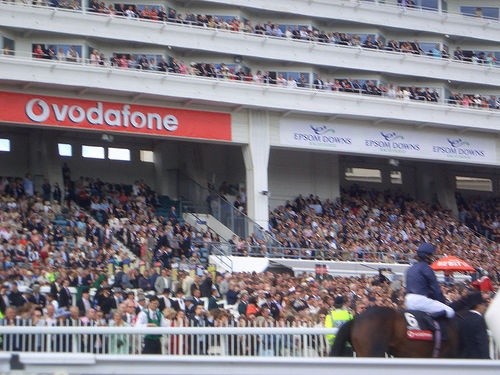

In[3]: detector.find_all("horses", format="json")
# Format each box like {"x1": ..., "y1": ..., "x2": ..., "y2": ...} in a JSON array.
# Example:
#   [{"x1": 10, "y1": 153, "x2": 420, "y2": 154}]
[{"x1": 328, "y1": 288, "x2": 500, "y2": 360}]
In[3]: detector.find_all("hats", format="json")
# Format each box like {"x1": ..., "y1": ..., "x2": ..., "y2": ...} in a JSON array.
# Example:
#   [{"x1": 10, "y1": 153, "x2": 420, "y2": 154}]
[
  {"x1": 162, "y1": 288, "x2": 171, "y2": 293},
  {"x1": 176, "y1": 288, "x2": 185, "y2": 294},
  {"x1": 477, "y1": 276, "x2": 495, "y2": 291},
  {"x1": 469, "y1": 292, "x2": 487, "y2": 308}
]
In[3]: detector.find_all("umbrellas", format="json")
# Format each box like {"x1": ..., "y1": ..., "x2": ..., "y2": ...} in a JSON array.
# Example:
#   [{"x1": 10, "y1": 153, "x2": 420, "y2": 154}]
[{"x1": 429, "y1": 256, "x2": 475, "y2": 271}]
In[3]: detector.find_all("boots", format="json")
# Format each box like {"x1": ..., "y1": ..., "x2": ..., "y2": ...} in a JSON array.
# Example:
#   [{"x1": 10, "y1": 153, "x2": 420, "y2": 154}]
[{"x1": 422, "y1": 310, "x2": 446, "y2": 329}]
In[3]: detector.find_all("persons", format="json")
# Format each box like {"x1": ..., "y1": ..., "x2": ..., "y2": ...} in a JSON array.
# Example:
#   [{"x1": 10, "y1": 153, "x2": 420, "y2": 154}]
[
  {"x1": 459, "y1": 292, "x2": 491, "y2": 359},
  {"x1": 0, "y1": 0, "x2": 500, "y2": 360},
  {"x1": 403, "y1": 243, "x2": 455, "y2": 331}
]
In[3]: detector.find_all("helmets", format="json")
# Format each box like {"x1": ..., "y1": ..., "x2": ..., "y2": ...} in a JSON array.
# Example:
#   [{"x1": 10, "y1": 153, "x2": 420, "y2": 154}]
[{"x1": 416, "y1": 242, "x2": 436, "y2": 256}]
